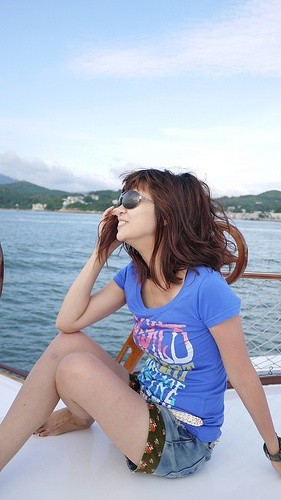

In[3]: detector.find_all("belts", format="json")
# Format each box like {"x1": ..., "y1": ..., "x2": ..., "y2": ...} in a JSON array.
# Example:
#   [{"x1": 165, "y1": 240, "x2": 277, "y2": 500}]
[{"x1": 139, "y1": 391, "x2": 220, "y2": 446}]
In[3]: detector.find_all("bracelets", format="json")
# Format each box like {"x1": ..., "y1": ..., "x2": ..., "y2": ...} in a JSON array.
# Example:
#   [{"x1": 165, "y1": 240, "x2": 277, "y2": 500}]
[{"x1": 263, "y1": 436, "x2": 281, "y2": 462}]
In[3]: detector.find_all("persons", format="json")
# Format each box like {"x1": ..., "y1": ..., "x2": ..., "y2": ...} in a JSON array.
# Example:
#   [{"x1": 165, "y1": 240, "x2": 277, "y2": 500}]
[{"x1": 0, "y1": 169, "x2": 281, "y2": 479}]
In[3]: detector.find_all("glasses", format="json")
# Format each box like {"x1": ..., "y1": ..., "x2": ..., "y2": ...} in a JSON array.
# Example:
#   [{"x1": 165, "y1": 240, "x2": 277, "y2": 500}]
[{"x1": 118, "y1": 190, "x2": 155, "y2": 209}]
